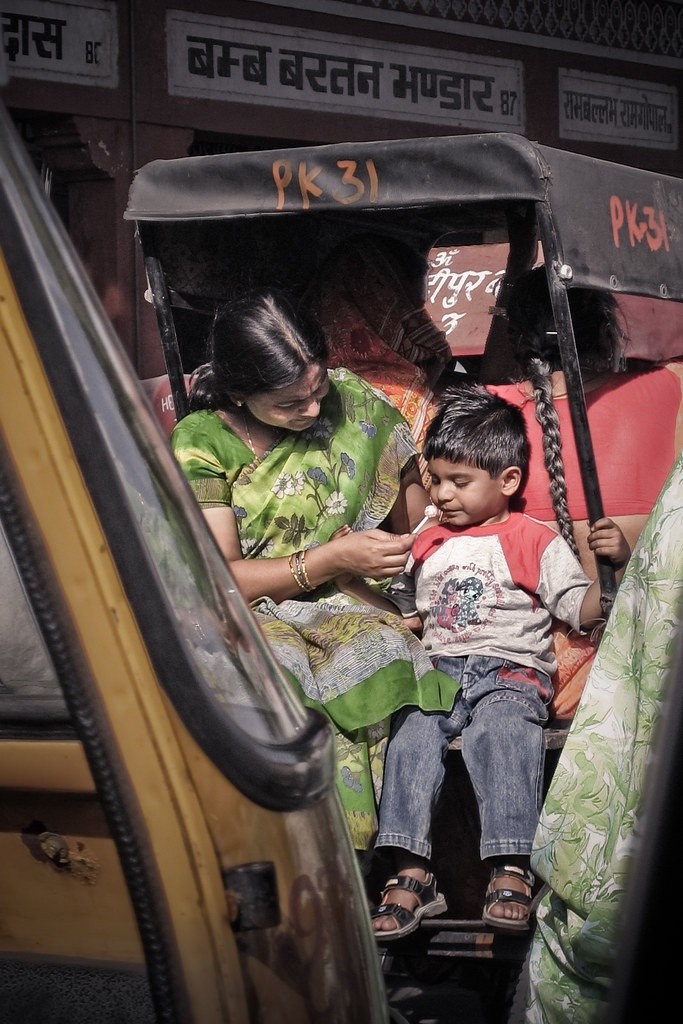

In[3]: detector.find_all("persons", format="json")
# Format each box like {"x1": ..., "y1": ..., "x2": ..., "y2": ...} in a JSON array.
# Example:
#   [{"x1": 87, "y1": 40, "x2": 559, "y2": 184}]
[
  {"x1": 169, "y1": 288, "x2": 462, "y2": 881},
  {"x1": 329, "y1": 386, "x2": 631, "y2": 943},
  {"x1": 291, "y1": 239, "x2": 453, "y2": 497},
  {"x1": 475, "y1": 289, "x2": 683, "y2": 566}
]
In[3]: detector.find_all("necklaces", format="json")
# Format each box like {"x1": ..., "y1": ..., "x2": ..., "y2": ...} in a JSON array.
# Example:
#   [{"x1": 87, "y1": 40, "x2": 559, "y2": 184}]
[{"x1": 243, "y1": 412, "x2": 259, "y2": 461}]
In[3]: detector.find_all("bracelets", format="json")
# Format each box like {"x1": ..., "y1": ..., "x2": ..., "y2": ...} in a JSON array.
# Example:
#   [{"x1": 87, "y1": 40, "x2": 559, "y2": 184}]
[{"x1": 288, "y1": 549, "x2": 316, "y2": 593}]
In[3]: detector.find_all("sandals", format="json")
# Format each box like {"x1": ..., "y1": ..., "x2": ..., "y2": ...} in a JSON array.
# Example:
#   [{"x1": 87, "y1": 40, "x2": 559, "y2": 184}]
[
  {"x1": 481, "y1": 862, "x2": 535, "y2": 931},
  {"x1": 370, "y1": 866, "x2": 448, "y2": 941}
]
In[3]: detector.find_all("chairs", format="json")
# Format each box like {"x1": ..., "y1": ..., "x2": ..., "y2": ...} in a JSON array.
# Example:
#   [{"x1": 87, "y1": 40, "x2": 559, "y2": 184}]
[{"x1": 307, "y1": 233, "x2": 453, "y2": 444}]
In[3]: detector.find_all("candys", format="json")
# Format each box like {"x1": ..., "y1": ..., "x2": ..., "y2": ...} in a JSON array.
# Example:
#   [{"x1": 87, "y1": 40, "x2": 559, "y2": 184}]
[{"x1": 424, "y1": 505, "x2": 438, "y2": 517}]
